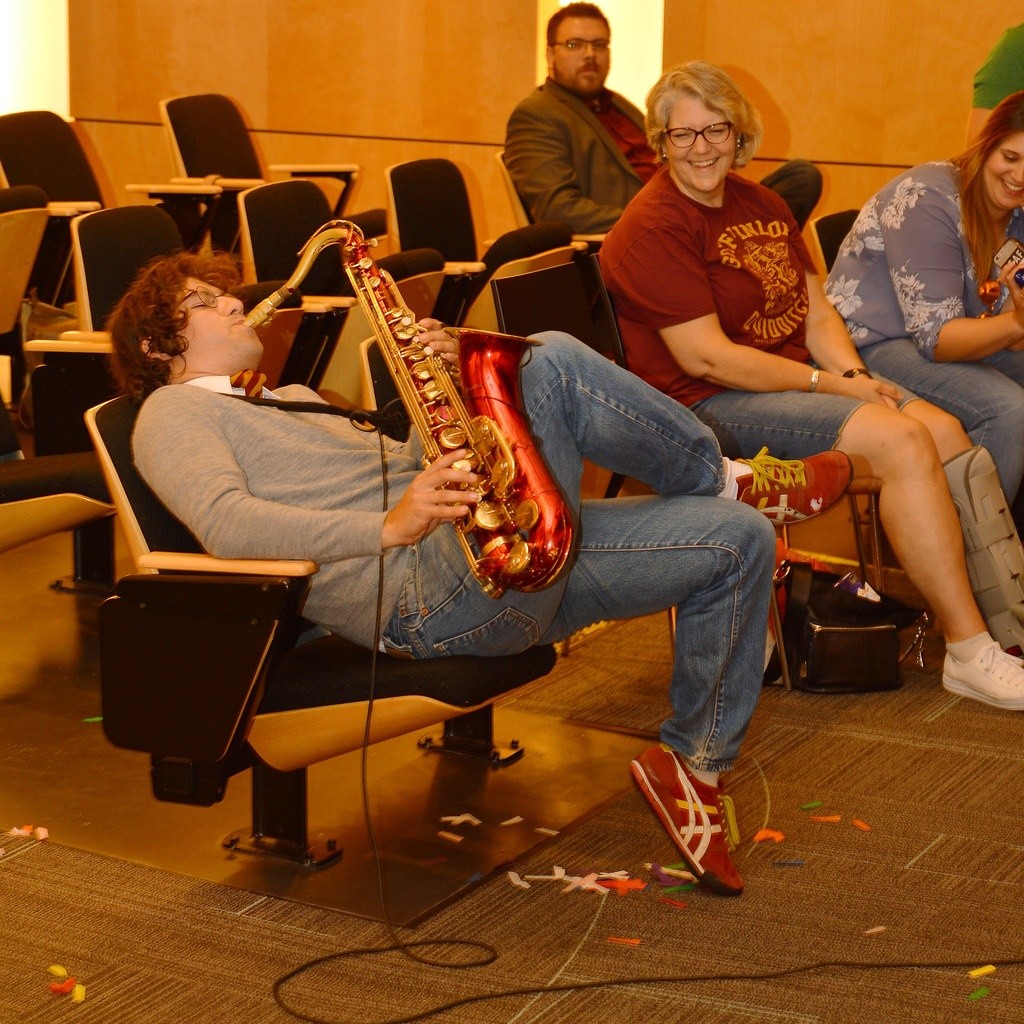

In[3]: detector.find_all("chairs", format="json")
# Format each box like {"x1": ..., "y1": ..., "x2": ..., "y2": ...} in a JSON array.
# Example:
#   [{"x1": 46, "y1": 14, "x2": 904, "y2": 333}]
[{"x1": 0, "y1": 93, "x2": 863, "y2": 868}]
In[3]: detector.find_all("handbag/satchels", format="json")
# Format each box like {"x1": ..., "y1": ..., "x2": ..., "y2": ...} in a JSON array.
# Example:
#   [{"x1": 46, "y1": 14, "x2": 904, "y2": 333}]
[{"x1": 762, "y1": 560, "x2": 929, "y2": 694}]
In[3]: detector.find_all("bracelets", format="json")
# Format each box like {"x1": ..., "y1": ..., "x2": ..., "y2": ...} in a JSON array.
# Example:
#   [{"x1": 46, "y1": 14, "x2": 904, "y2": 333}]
[
  {"x1": 842, "y1": 368, "x2": 874, "y2": 379},
  {"x1": 809, "y1": 369, "x2": 820, "y2": 392}
]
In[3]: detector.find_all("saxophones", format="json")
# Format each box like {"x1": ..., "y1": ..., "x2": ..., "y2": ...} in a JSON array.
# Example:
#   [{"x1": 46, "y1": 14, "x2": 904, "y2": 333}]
[{"x1": 240, "y1": 220, "x2": 575, "y2": 601}]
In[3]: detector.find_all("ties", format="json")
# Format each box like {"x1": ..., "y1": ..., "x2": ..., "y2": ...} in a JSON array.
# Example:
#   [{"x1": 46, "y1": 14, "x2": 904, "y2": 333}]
[{"x1": 230, "y1": 368, "x2": 267, "y2": 399}]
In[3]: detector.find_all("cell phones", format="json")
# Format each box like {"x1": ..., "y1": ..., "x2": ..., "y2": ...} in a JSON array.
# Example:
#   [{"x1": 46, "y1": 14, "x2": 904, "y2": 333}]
[{"x1": 992, "y1": 238, "x2": 1024, "y2": 274}]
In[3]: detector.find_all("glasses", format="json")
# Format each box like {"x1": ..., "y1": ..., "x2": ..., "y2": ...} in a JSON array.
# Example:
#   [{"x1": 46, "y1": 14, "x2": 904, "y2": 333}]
[
  {"x1": 174, "y1": 286, "x2": 238, "y2": 308},
  {"x1": 552, "y1": 39, "x2": 612, "y2": 53},
  {"x1": 661, "y1": 121, "x2": 732, "y2": 147}
]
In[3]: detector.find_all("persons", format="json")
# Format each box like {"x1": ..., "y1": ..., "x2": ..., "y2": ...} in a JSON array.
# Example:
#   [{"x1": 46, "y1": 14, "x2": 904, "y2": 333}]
[
  {"x1": 599, "y1": 62, "x2": 1024, "y2": 712},
  {"x1": 963, "y1": 21, "x2": 1024, "y2": 249},
  {"x1": 104, "y1": 248, "x2": 852, "y2": 897},
  {"x1": 499, "y1": 3, "x2": 823, "y2": 234},
  {"x1": 824, "y1": 92, "x2": 1024, "y2": 551}
]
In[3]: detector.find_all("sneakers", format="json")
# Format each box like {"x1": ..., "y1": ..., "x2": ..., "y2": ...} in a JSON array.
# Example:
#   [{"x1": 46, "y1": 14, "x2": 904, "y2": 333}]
[
  {"x1": 628, "y1": 742, "x2": 744, "y2": 896},
  {"x1": 942, "y1": 641, "x2": 1024, "y2": 711},
  {"x1": 735, "y1": 446, "x2": 854, "y2": 525}
]
